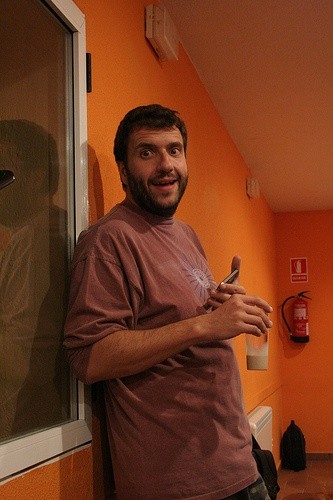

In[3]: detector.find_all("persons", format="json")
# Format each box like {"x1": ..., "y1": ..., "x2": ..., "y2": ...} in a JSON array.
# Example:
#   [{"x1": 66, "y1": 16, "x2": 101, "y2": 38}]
[
  {"x1": 0, "y1": 118, "x2": 69, "y2": 442},
  {"x1": 64, "y1": 103, "x2": 274, "y2": 500}
]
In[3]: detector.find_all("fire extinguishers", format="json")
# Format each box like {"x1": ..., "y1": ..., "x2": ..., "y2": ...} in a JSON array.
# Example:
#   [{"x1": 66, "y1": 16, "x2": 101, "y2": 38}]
[{"x1": 280, "y1": 287, "x2": 315, "y2": 345}]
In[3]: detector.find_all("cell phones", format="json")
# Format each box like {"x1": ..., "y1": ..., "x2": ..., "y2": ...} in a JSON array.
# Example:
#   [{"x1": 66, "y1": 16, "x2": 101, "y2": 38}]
[
  {"x1": 203, "y1": 268, "x2": 239, "y2": 310},
  {"x1": 0, "y1": 170, "x2": 16, "y2": 190}
]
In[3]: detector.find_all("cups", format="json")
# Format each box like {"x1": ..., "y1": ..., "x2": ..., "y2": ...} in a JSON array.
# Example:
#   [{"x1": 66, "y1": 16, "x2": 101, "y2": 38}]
[{"x1": 246, "y1": 307, "x2": 269, "y2": 370}]
